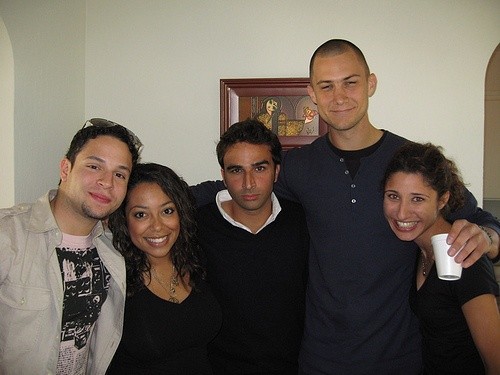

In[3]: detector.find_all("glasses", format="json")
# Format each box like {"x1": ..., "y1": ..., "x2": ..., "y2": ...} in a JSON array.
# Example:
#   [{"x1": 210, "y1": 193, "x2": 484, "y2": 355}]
[{"x1": 80, "y1": 118, "x2": 143, "y2": 153}]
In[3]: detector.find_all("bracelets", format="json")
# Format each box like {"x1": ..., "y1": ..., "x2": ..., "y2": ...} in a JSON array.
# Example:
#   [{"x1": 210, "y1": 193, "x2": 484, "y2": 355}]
[{"x1": 479, "y1": 226, "x2": 493, "y2": 245}]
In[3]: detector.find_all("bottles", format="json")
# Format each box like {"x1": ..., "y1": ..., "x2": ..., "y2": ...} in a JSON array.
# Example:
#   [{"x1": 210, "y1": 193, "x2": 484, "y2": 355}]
[{"x1": 431, "y1": 233, "x2": 462, "y2": 280}]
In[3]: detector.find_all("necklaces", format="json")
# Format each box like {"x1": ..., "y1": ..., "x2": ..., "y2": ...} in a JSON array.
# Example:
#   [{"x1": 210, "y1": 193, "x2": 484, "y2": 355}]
[
  {"x1": 146, "y1": 258, "x2": 181, "y2": 304},
  {"x1": 421, "y1": 257, "x2": 430, "y2": 276}
]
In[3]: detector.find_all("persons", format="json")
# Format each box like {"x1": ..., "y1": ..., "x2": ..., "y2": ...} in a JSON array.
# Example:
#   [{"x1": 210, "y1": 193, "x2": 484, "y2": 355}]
[
  {"x1": 381, "y1": 142, "x2": 499, "y2": 374},
  {"x1": 1, "y1": 119, "x2": 144, "y2": 375},
  {"x1": 106, "y1": 162, "x2": 223, "y2": 375},
  {"x1": 188, "y1": 39, "x2": 500, "y2": 374},
  {"x1": 195, "y1": 120, "x2": 312, "y2": 375}
]
